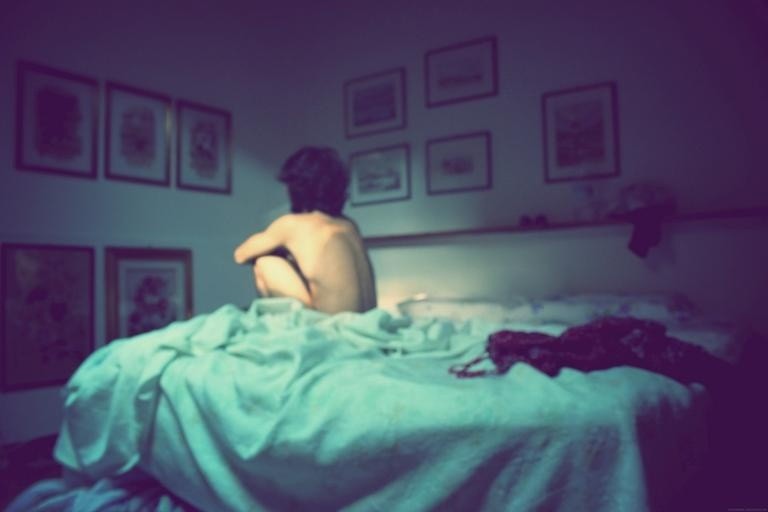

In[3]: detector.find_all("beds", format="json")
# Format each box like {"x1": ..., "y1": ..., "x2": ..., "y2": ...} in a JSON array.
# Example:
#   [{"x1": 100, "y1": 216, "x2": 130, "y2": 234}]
[{"x1": 67, "y1": 209, "x2": 766, "y2": 512}]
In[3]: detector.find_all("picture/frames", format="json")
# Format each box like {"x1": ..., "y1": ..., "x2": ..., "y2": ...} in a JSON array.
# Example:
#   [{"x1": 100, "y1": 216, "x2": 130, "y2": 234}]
[
  {"x1": 101, "y1": 81, "x2": 174, "y2": 187},
  {"x1": 541, "y1": 82, "x2": 618, "y2": 185},
  {"x1": 348, "y1": 143, "x2": 412, "y2": 204},
  {"x1": 175, "y1": 100, "x2": 232, "y2": 195},
  {"x1": 423, "y1": 36, "x2": 498, "y2": 109},
  {"x1": 12, "y1": 58, "x2": 99, "y2": 180},
  {"x1": 105, "y1": 244, "x2": 196, "y2": 345},
  {"x1": 346, "y1": 67, "x2": 407, "y2": 138},
  {"x1": 424, "y1": 130, "x2": 492, "y2": 196},
  {"x1": 3, "y1": 239, "x2": 95, "y2": 391}
]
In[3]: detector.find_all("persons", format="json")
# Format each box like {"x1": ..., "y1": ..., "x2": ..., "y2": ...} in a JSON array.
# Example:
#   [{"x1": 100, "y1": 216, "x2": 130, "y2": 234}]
[{"x1": 234, "y1": 144, "x2": 380, "y2": 318}]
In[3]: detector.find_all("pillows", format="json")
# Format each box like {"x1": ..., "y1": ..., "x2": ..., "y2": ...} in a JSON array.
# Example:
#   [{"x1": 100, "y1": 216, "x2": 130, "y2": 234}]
[{"x1": 397, "y1": 291, "x2": 696, "y2": 325}]
